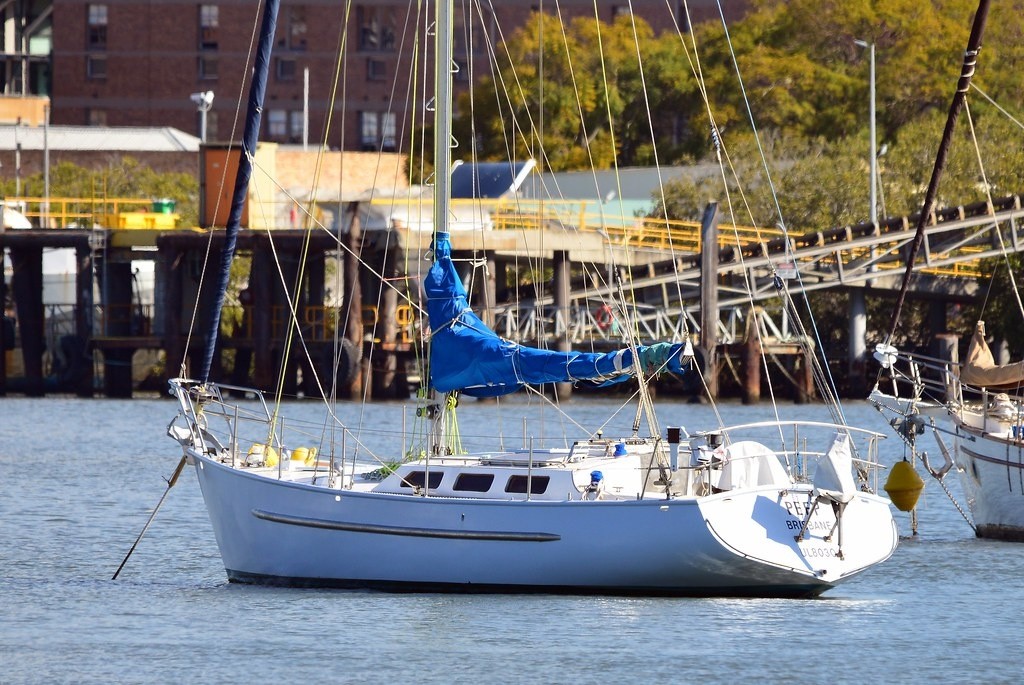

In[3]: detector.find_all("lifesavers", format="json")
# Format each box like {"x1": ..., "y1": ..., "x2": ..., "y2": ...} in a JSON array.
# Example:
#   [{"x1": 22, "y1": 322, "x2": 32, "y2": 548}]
[{"x1": 597, "y1": 306, "x2": 613, "y2": 330}]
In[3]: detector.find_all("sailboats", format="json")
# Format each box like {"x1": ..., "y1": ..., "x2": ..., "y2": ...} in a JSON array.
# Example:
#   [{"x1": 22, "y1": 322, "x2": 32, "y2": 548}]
[
  {"x1": 861, "y1": 1, "x2": 1024, "y2": 549},
  {"x1": 164, "y1": 0, "x2": 902, "y2": 600}
]
